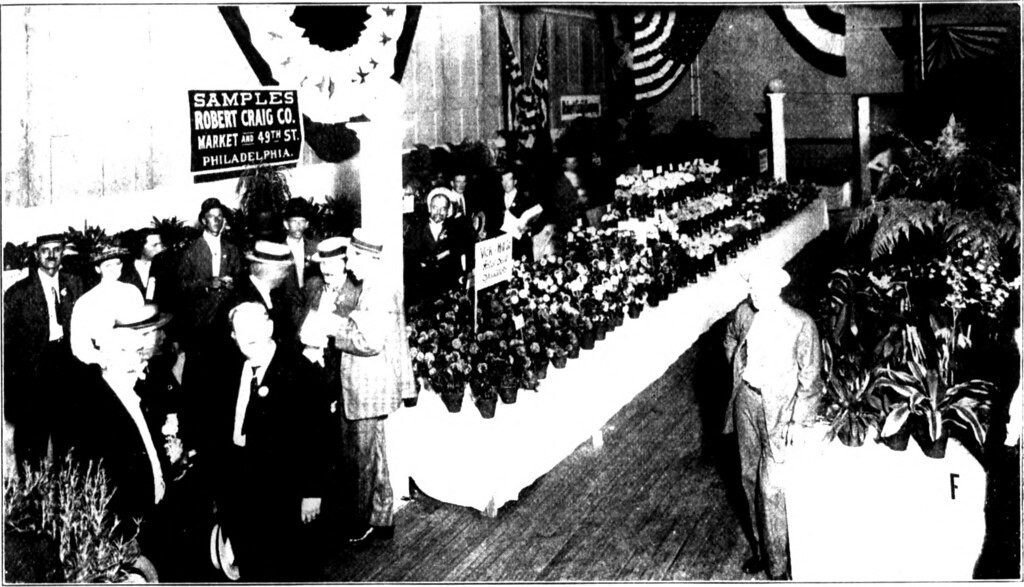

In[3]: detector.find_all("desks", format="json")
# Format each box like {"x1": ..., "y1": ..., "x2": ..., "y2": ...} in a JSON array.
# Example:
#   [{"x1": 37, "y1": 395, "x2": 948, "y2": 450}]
[{"x1": 391, "y1": 184, "x2": 832, "y2": 519}]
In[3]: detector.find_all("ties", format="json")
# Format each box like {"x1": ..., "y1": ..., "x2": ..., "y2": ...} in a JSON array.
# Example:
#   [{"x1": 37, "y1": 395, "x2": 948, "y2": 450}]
[{"x1": 240, "y1": 365, "x2": 258, "y2": 435}]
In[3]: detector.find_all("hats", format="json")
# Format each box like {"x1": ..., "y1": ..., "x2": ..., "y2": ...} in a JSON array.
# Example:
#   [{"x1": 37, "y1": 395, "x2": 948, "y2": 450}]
[
  {"x1": 26, "y1": 234, "x2": 70, "y2": 252},
  {"x1": 309, "y1": 236, "x2": 350, "y2": 261},
  {"x1": 199, "y1": 198, "x2": 232, "y2": 226},
  {"x1": 93, "y1": 248, "x2": 127, "y2": 264},
  {"x1": 344, "y1": 229, "x2": 389, "y2": 260},
  {"x1": 113, "y1": 304, "x2": 172, "y2": 329},
  {"x1": 744, "y1": 263, "x2": 790, "y2": 288},
  {"x1": 246, "y1": 241, "x2": 297, "y2": 266},
  {"x1": 281, "y1": 197, "x2": 315, "y2": 224},
  {"x1": 426, "y1": 186, "x2": 455, "y2": 218}
]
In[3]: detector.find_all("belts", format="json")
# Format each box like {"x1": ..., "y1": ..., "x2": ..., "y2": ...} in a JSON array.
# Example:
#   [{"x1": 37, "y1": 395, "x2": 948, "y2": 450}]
[{"x1": 743, "y1": 380, "x2": 761, "y2": 397}]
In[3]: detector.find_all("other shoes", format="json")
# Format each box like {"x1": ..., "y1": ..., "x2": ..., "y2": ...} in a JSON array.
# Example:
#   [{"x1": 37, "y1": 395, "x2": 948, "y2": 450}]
[{"x1": 743, "y1": 557, "x2": 765, "y2": 574}]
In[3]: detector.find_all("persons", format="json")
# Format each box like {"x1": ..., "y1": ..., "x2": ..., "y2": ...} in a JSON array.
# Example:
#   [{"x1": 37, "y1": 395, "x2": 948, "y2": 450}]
[
  {"x1": 723, "y1": 256, "x2": 821, "y2": 581},
  {"x1": 400, "y1": 129, "x2": 609, "y2": 315},
  {"x1": 4, "y1": 194, "x2": 417, "y2": 584}
]
